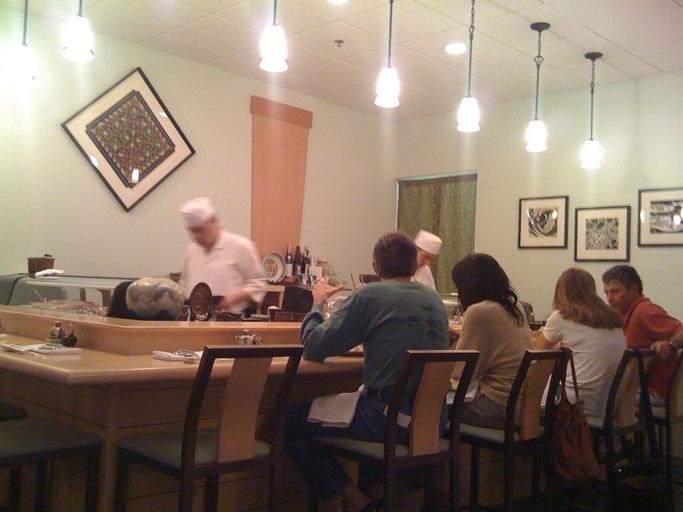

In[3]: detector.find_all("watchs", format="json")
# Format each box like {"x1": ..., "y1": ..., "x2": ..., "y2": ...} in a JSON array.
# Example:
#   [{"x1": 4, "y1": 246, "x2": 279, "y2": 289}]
[{"x1": 667, "y1": 339, "x2": 680, "y2": 352}]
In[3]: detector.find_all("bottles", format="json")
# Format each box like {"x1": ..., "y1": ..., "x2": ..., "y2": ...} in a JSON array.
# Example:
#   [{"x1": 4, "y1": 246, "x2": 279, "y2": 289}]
[
  {"x1": 49, "y1": 320, "x2": 62, "y2": 338},
  {"x1": 285, "y1": 244, "x2": 310, "y2": 277}
]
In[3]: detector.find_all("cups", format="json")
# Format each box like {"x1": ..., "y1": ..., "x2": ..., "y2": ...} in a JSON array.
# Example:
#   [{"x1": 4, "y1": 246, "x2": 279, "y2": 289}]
[{"x1": 325, "y1": 278, "x2": 345, "y2": 320}]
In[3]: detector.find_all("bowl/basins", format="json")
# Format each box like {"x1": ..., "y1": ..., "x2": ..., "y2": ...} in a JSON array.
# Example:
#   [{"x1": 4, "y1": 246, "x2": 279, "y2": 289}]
[{"x1": 262, "y1": 251, "x2": 286, "y2": 283}]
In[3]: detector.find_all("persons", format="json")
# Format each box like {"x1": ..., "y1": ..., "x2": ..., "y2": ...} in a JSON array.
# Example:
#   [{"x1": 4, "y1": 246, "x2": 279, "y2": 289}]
[
  {"x1": 180, "y1": 196, "x2": 268, "y2": 318},
  {"x1": 445, "y1": 254, "x2": 536, "y2": 432},
  {"x1": 282, "y1": 233, "x2": 449, "y2": 512},
  {"x1": 415, "y1": 229, "x2": 444, "y2": 291},
  {"x1": 538, "y1": 269, "x2": 631, "y2": 506},
  {"x1": 602, "y1": 266, "x2": 683, "y2": 492}
]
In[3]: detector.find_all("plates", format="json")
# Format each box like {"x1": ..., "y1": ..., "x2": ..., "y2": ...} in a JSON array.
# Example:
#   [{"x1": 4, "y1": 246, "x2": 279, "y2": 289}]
[{"x1": 47, "y1": 339, "x2": 62, "y2": 343}]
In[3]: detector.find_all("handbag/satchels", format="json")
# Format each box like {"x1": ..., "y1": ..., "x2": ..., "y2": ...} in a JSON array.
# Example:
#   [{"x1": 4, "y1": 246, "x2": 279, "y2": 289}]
[{"x1": 544, "y1": 398, "x2": 600, "y2": 481}]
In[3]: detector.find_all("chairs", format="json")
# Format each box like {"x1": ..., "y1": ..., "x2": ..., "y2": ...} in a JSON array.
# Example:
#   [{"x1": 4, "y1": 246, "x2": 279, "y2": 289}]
[
  {"x1": 0, "y1": 416, "x2": 105, "y2": 511},
  {"x1": 309, "y1": 350, "x2": 481, "y2": 512},
  {"x1": 112, "y1": 343, "x2": 305, "y2": 512},
  {"x1": 636, "y1": 345, "x2": 682, "y2": 511},
  {"x1": 544, "y1": 348, "x2": 657, "y2": 511},
  {"x1": 0, "y1": 399, "x2": 26, "y2": 421},
  {"x1": 443, "y1": 348, "x2": 572, "y2": 511}
]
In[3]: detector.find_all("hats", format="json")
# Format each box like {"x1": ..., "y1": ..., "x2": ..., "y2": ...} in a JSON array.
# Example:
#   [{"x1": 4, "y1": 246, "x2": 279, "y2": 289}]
[
  {"x1": 414, "y1": 229, "x2": 442, "y2": 256},
  {"x1": 179, "y1": 199, "x2": 216, "y2": 224}
]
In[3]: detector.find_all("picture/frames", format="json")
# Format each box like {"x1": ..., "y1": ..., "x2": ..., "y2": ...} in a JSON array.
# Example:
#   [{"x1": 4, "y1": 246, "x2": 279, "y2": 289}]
[
  {"x1": 61, "y1": 65, "x2": 196, "y2": 212},
  {"x1": 575, "y1": 206, "x2": 631, "y2": 262},
  {"x1": 638, "y1": 187, "x2": 683, "y2": 247},
  {"x1": 518, "y1": 195, "x2": 569, "y2": 249}
]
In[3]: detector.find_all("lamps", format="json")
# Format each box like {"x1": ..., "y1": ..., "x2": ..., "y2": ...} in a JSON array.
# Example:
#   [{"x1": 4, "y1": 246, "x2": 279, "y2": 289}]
[
  {"x1": 456, "y1": 1, "x2": 481, "y2": 134},
  {"x1": 257, "y1": 0, "x2": 289, "y2": 73},
  {"x1": 60, "y1": 0, "x2": 94, "y2": 67},
  {"x1": 373, "y1": 1, "x2": 400, "y2": 108},
  {"x1": 580, "y1": 53, "x2": 604, "y2": 172},
  {"x1": 523, "y1": 22, "x2": 550, "y2": 153}
]
